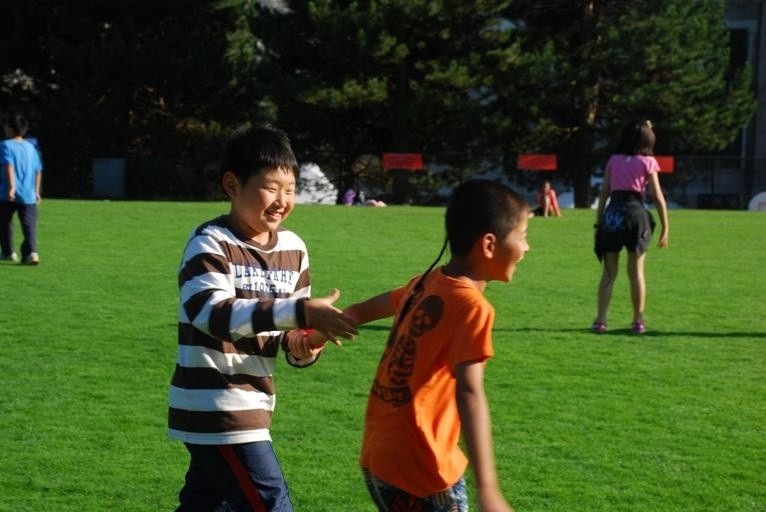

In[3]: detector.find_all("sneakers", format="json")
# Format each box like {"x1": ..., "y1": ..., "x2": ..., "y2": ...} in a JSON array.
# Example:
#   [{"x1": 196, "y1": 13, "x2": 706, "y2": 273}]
[
  {"x1": 21, "y1": 252, "x2": 39, "y2": 265},
  {"x1": 631, "y1": 321, "x2": 647, "y2": 334},
  {"x1": 0, "y1": 251, "x2": 18, "y2": 262},
  {"x1": 592, "y1": 321, "x2": 607, "y2": 333}
]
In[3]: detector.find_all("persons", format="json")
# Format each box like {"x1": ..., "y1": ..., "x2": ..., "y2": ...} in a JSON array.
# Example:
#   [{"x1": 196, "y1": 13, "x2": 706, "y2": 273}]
[
  {"x1": 0, "y1": 114, "x2": 44, "y2": 265},
  {"x1": 591, "y1": 120, "x2": 669, "y2": 333},
  {"x1": 168, "y1": 122, "x2": 359, "y2": 512},
  {"x1": 302, "y1": 176, "x2": 530, "y2": 512},
  {"x1": 528, "y1": 180, "x2": 562, "y2": 218}
]
